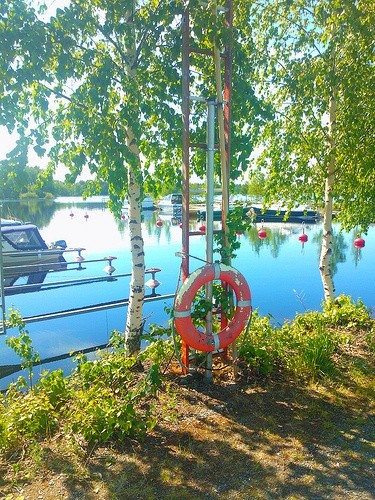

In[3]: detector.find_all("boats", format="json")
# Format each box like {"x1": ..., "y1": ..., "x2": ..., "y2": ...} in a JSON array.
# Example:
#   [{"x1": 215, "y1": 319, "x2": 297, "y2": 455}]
[{"x1": 0, "y1": 218, "x2": 67, "y2": 275}]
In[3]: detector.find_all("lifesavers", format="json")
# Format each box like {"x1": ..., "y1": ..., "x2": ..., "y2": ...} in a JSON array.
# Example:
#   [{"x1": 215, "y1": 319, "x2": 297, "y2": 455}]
[{"x1": 173, "y1": 263, "x2": 252, "y2": 352}]
